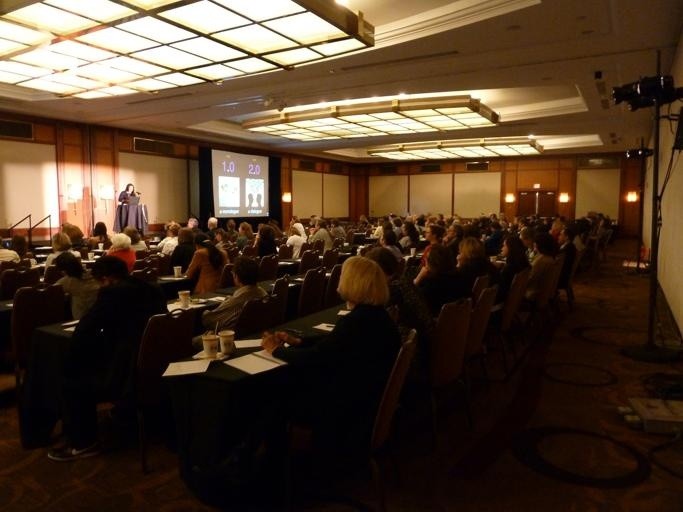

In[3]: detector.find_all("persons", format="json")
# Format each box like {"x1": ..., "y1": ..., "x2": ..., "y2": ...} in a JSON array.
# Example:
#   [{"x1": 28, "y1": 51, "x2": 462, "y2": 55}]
[
  {"x1": 271, "y1": 223, "x2": 284, "y2": 240},
  {"x1": 119, "y1": 184, "x2": 138, "y2": 205},
  {"x1": 44, "y1": 232, "x2": 81, "y2": 279},
  {"x1": 192, "y1": 258, "x2": 269, "y2": 347},
  {"x1": 405, "y1": 225, "x2": 447, "y2": 286},
  {"x1": 247, "y1": 193, "x2": 262, "y2": 210},
  {"x1": 46, "y1": 256, "x2": 159, "y2": 461},
  {"x1": 205, "y1": 217, "x2": 222, "y2": 244},
  {"x1": 168, "y1": 228, "x2": 205, "y2": 272},
  {"x1": 261, "y1": 257, "x2": 401, "y2": 420},
  {"x1": 560, "y1": 227, "x2": 579, "y2": 290},
  {"x1": 52, "y1": 252, "x2": 101, "y2": 318},
  {"x1": 62, "y1": 224, "x2": 84, "y2": 247},
  {"x1": 290, "y1": 216, "x2": 307, "y2": 239},
  {"x1": 308, "y1": 220, "x2": 333, "y2": 255},
  {"x1": 520, "y1": 227, "x2": 574, "y2": 302},
  {"x1": 123, "y1": 225, "x2": 147, "y2": 251},
  {"x1": 484, "y1": 236, "x2": 532, "y2": 335},
  {"x1": 104, "y1": 233, "x2": 136, "y2": 272},
  {"x1": 286, "y1": 227, "x2": 307, "y2": 259},
  {"x1": 188, "y1": 217, "x2": 209, "y2": 240},
  {"x1": 227, "y1": 219, "x2": 239, "y2": 241},
  {"x1": 235, "y1": 223, "x2": 254, "y2": 249},
  {"x1": 380, "y1": 229, "x2": 406, "y2": 262},
  {"x1": 243, "y1": 224, "x2": 275, "y2": 258},
  {"x1": 525, "y1": 234, "x2": 561, "y2": 299},
  {"x1": 186, "y1": 234, "x2": 227, "y2": 295},
  {"x1": 87, "y1": 222, "x2": 112, "y2": 251},
  {"x1": 215, "y1": 228, "x2": 242, "y2": 265},
  {"x1": 364, "y1": 247, "x2": 434, "y2": 342},
  {"x1": 10, "y1": 236, "x2": 39, "y2": 265},
  {"x1": 309, "y1": 215, "x2": 318, "y2": 226},
  {"x1": 349, "y1": 213, "x2": 609, "y2": 257},
  {"x1": 0, "y1": 240, "x2": 21, "y2": 263},
  {"x1": 157, "y1": 221, "x2": 182, "y2": 256},
  {"x1": 456, "y1": 237, "x2": 483, "y2": 272},
  {"x1": 330, "y1": 221, "x2": 347, "y2": 246}
]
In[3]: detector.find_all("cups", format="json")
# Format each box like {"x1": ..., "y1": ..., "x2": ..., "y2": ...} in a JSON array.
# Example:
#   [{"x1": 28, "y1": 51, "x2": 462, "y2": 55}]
[
  {"x1": 489, "y1": 256, "x2": 496, "y2": 263},
  {"x1": 173, "y1": 266, "x2": 181, "y2": 278},
  {"x1": 155, "y1": 238, "x2": 161, "y2": 242},
  {"x1": 410, "y1": 248, "x2": 416, "y2": 257},
  {"x1": 202, "y1": 334, "x2": 219, "y2": 357},
  {"x1": 87, "y1": 253, "x2": 94, "y2": 261},
  {"x1": 218, "y1": 330, "x2": 235, "y2": 355},
  {"x1": 2, "y1": 238, "x2": 14, "y2": 249},
  {"x1": 178, "y1": 290, "x2": 190, "y2": 308},
  {"x1": 98, "y1": 244, "x2": 103, "y2": 250}
]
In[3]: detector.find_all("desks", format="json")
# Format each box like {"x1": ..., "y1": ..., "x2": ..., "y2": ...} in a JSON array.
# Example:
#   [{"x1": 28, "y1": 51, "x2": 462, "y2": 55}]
[
  {"x1": 155, "y1": 311, "x2": 351, "y2": 512},
  {"x1": 157, "y1": 274, "x2": 187, "y2": 299},
  {"x1": 38, "y1": 293, "x2": 269, "y2": 449},
  {"x1": 338, "y1": 253, "x2": 351, "y2": 264},
  {"x1": 281, "y1": 256, "x2": 325, "y2": 274},
  {"x1": 278, "y1": 261, "x2": 299, "y2": 277}
]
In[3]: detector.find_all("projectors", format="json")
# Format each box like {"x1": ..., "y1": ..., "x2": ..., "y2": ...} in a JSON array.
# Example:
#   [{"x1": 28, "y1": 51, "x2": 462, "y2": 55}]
[
  {"x1": 611, "y1": 75, "x2": 675, "y2": 111},
  {"x1": 624, "y1": 148, "x2": 653, "y2": 160}
]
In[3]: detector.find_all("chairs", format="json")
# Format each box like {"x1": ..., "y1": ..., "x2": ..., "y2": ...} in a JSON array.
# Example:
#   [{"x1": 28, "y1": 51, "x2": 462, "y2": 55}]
[
  {"x1": 313, "y1": 239, "x2": 325, "y2": 255},
  {"x1": 559, "y1": 251, "x2": 583, "y2": 306},
  {"x1": 230, "y1": 300, "x2": 262, "y2": 339},
  {"x1": 332, "y1": 237, "x2": 344, "y2": 251},
  {"x1": 472, "y1": 288, "x2": 495, "y2": 353},
  {"x1": 148, "y1": 254, "x2": 170, "y2": 268},
  {"x1": 276, "y1": 244, "x2": 293, "y2": 259},
  {"x1": 542, "y1": 263, "x2": 559, "y2": 304},
  {"x1": 322, "y1": 248, "x2": 339, "y2": 270},
  {"x1": 435, "y1": 298, "x2": 472, "y2": 343},
  {"x1": 219, "y1": 264, "x2": 235, "y2": 289},
  {"x1": 14, "y1": 288, "x2": 45, "y2": 394},
  {"x1": 301, "y1": 250, "x2": 320, "y2": 275},
  {"x1": 505, "y1": 272, "x2": 528, "y2": 327},
  {"x1": 256, "y1": 254, "x2": 279, "y2": 282},
  {"x1": 471, "y1": 274, "x2": 489, "y2": 309},
  {"x1": 298, "y1": 267, "x2": 326, "y2": 318},
  {"x1": 370, "y1": 329, "x2": 417, "y2": 448},
  {"x1": 299, "y1": 242, "x2": 314, "y2": 258},
  {"x1": 140, "y1": 309, "x2": 184, "y2": 368},
  {"x1": 44, "y1": 266, "x2": 62, "y2": 286},
  {"x1": 0, "y1": 269, "x2": 26, "y2": 301},
  {"x1": 327, "y1": 264, "x2": 342, "y2": 309},
  {"x1": 349, "y1": 233, "x2": 366, "y2": 256},
  {"x1": 135, "y1": 250, "x2": 145, "y2": 259}
]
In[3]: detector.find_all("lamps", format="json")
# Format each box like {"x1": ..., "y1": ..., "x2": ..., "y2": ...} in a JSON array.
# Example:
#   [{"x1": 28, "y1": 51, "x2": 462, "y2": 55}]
[
  {"x1": 559, "y1": 192, "x2": 571, "y2": 210},
  {"x1": 628, "y1": 190, "x2": 637, "y2": 209}
]
